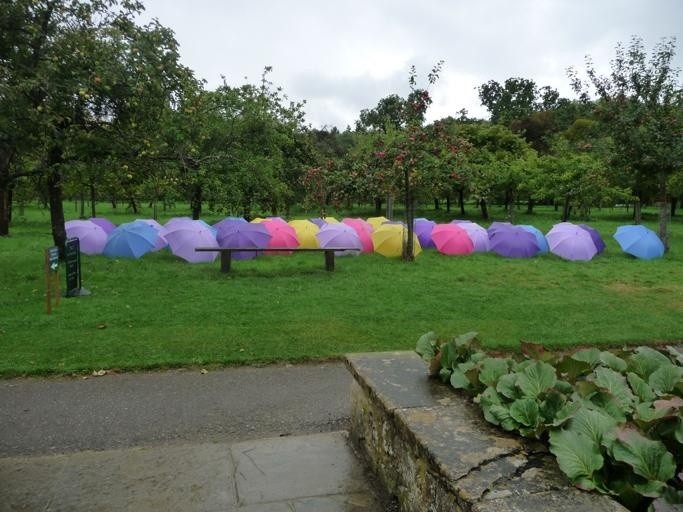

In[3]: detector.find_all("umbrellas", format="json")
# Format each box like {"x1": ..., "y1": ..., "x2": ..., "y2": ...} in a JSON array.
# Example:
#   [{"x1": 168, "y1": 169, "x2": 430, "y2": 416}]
[{"x1": 614, "y1": 223, "x2": 664, "y2": 257}]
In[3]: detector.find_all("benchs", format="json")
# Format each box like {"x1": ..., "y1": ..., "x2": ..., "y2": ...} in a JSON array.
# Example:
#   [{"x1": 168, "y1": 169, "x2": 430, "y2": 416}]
[{"x1": 194, "y1": 248, "x2": 360, "y2": 273}]
